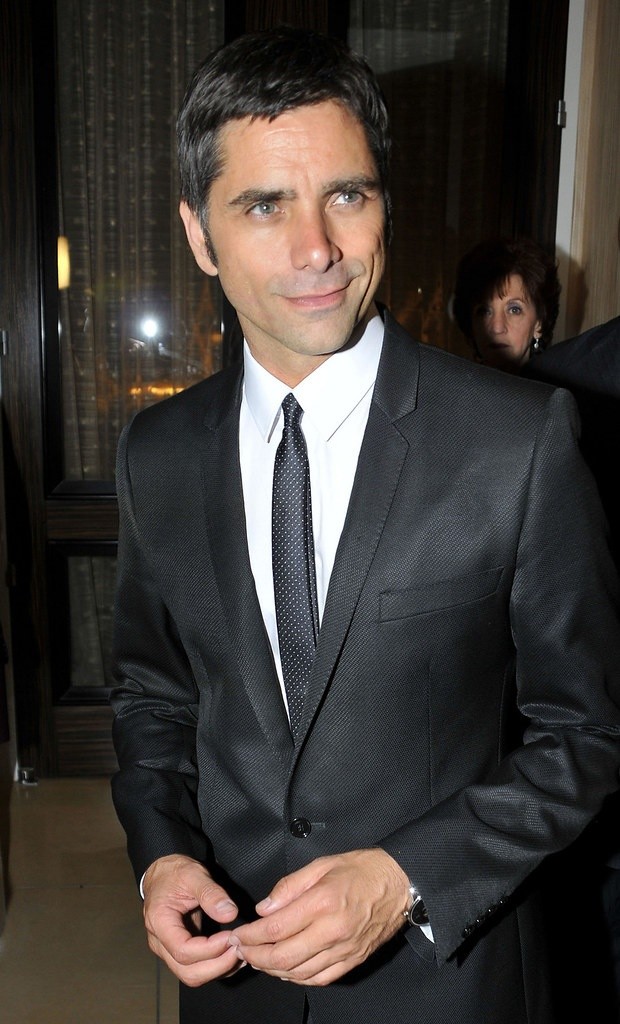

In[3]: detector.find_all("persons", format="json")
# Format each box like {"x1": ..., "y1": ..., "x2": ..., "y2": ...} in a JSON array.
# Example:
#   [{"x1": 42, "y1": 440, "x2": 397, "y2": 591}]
[
  {"x1": 107, "y1": 26, "x2": 620, "y2": 1023},
  {"x1": 453, "y1": 236, "x2": 620, "y2": 1024}
]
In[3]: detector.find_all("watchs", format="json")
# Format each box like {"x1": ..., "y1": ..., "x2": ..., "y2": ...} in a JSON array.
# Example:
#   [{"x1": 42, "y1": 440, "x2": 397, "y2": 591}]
[{"x1": 403, "y1": 872, "x2": 429, "y2": 927}]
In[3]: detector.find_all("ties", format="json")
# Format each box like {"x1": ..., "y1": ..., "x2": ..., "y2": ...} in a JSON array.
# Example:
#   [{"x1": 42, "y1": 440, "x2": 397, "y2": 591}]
[{"x1": 272, "y1": 393, "x2": 320, "y2": 746}]
